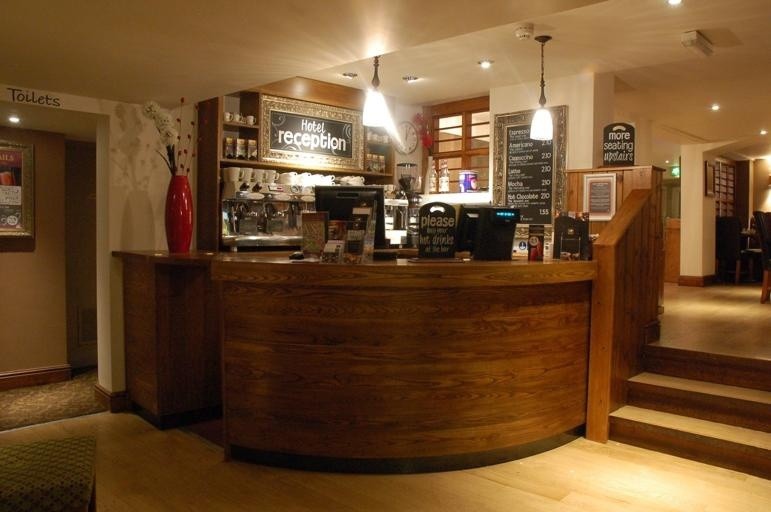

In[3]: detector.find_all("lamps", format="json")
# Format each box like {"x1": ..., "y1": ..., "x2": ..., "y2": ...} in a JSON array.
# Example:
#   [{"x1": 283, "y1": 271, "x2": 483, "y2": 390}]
[
  {"x1": 681, "y1": 30, "x2": 713, "y2": 57},
  {"x1": 362, "y1": 56, "x2": 390, "y2": 127},
  {"x1": 530, "y1": 36, "x2": 554, "y2": 141}
]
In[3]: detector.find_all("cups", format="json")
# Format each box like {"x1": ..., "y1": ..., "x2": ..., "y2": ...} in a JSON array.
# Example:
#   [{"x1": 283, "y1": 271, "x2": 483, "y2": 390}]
[
  {"x1": 222, "y1": 111, "x2": 257, "y2": 126},
  {"x1": 222, "y1": 166, "x2": 369, "y2": 187}
]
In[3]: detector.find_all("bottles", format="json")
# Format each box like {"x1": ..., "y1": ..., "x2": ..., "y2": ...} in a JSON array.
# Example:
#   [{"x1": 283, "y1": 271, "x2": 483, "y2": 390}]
[
  {"x1": 222, "y1": 137, "x2": 258, "y2": 161},
  {"x1": 429, "y1": 160, "x2": 439, "y2": 193},
  {"x1": 438, "y1": 159, "x2": 450, "y2": 193}
]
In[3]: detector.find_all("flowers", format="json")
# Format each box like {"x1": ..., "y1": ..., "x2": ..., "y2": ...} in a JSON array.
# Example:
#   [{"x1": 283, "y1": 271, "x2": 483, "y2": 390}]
[
  {"x1": 413, "y1": 113, "x2": 434, "y2": 156},
  {"x1": 143, "y1": 97, "x2": 198, "y2": 176}
]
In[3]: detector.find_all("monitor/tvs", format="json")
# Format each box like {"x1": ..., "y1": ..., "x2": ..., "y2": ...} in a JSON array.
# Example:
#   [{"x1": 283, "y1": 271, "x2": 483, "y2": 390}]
[
  {"x1": 455, "y1": 204, "x2": 521, "y2": 260},
  {"x1": 314, "y1": 185, "x2": 386, "y2": 252}
]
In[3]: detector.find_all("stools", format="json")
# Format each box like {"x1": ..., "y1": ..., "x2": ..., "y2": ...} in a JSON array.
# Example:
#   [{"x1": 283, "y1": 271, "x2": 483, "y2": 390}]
[{"x1": 0, "y1": 436, "x2": 96, "y2": 512}]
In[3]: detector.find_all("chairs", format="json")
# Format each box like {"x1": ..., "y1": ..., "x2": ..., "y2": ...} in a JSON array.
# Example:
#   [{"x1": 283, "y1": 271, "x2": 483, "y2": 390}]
[{"x1": 716, "y1": 211, "x2": 771, "y2": 304}]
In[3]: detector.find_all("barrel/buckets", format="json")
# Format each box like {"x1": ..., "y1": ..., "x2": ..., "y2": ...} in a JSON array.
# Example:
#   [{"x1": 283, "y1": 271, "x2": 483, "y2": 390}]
[{"x1": 458, "y1": 170, "x2": 479, "y2": 192}]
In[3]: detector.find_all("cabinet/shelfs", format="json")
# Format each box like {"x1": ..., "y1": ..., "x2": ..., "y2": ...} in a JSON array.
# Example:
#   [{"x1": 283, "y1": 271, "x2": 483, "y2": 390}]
[{"x1": 197, "y1": 90, "x2": 396, "y2": 252}]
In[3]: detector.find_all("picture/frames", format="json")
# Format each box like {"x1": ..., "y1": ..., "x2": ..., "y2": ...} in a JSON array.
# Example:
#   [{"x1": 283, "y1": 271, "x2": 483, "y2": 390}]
[
  {"x1": 705, "y1": 160, "x2": 715, "y2": 197},
  {"x1": 0, "y1": 139, "x2": 34, "y2": 239}
]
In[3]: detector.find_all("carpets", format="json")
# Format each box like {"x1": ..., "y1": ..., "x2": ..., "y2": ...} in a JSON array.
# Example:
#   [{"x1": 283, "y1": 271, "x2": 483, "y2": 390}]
[{"x1": 0, "y1": 370, "x2": 107, "y2": 431}]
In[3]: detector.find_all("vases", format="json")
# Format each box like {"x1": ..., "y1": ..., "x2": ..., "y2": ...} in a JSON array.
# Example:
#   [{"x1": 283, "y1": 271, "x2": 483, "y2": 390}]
[
  {"x1": 425, "y1": 156, "x2": 433, "y2": 194},
  {"x1": 165, "y1": 176, "x2": 193, "y2": 253}
]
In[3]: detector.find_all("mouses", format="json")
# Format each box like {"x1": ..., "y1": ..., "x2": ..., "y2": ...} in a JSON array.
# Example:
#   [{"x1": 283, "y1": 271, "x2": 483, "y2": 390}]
[{"x1": 289, "y1": 252, "x2": 304, "y2": 259}]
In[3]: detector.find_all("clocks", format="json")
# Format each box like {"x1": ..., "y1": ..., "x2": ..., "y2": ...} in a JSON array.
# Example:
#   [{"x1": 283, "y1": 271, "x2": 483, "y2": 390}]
[{"x1": 395, "y1": 121, "x2": 418, "y2": 155}]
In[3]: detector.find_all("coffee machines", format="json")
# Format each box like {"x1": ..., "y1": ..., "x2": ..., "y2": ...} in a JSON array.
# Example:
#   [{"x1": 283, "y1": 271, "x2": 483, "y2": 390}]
[{"x1": 395, "y1": 162, "x2": 425, "y2": 224}]
